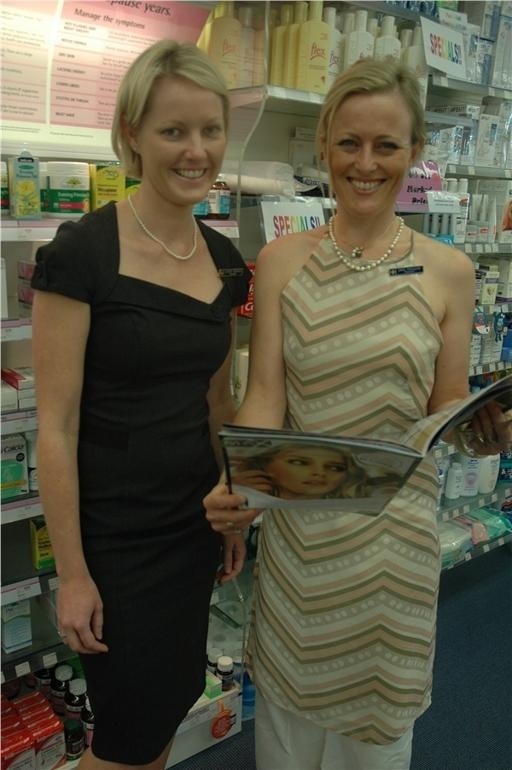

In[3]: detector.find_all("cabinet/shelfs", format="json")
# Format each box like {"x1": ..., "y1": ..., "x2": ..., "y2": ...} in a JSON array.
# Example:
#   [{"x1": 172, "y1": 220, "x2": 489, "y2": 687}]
[{"x1": 0, "y1": 3, "x2": 511, "y2": 766}]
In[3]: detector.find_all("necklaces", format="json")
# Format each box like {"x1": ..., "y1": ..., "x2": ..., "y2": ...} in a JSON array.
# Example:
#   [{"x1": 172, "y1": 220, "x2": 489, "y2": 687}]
[
  {"x1": 327, "y1": 210, "x2": 405, "y2": 271},
  {"x1": 128, "y1": 191, "x2": 197, "y2": 260},
  {"x1": 350, "y1": 220, "x2": 395, "y2": 258}
]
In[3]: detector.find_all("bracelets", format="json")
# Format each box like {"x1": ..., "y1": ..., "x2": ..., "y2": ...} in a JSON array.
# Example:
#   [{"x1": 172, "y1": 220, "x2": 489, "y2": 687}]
[{"x1": 452, "y1": 429, "x2": 488, "y2": 458}]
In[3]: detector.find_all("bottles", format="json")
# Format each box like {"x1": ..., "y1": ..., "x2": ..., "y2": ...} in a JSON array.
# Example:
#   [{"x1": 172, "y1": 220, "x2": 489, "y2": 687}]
[
  {"x1": 191, "y1": 173, "x2": 230, "y2": 220},
  {"x1": 204, "y1": 638, "x2": 238, "y2": 694},
  {"x1": 428, "y1": 454, "x2": 501, "y2": 506},
  {"x1": 33, "y1": 655, "x2": 102, "y2": 762},
  {"x1": 1, "y1": 142, "x2": 90, "y2": 219},
  {"x1": 422, "y1": 178, "x2": 470, "y2": 245},
  {"x1": 188, "y1": 0, "x2": 429, "y2": 122}
]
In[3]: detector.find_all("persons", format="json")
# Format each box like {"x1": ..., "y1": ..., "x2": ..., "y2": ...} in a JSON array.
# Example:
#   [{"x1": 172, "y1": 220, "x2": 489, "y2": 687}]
[
  {"x1": 235, "y1": 441, "x2": 376, "y2": 501},
  {"x1": 199, "y1": 56, "x2": 512, "y2": 769},
  {"x1": 30, "y1": 38, "x2": 253, "y2": 770}
]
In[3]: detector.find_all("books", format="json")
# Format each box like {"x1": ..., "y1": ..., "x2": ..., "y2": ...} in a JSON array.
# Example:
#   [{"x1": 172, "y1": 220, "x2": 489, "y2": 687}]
[{"x1": 217, "y1": 373, "x2": 512, "y2": 518}]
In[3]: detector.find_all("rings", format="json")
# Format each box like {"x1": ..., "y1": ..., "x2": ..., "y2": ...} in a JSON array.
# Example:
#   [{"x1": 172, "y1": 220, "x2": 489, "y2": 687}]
[
  {"x1": 58, "y1": 631, "x2": 67, "y2": 638},
  {"x1": 235, "y1": 526, "x2": 242, "y2": 535},
  {"x1": 227, "y1": 521, "x2": 235, "y2": 529},
  {"x1": 477, "y1": 432, "x2": 496, "y2": 447}
]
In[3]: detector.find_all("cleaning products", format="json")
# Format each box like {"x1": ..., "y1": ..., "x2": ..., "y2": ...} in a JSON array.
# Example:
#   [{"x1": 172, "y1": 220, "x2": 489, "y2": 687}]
[
  {"x1": 436, "y1": 449, "x2": 500, "y2": 498},
  {"x1": 423, "y1": 178, "x2": 470, "y2": 244},
  {"x1": 203, "y1": 1, "x2": 429, "y2": 112}
]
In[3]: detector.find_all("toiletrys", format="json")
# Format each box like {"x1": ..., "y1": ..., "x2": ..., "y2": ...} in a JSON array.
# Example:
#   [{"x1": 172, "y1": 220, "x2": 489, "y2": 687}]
[{"x1": 470, "y1": 194, "x2": 497, "y2": 242}]
